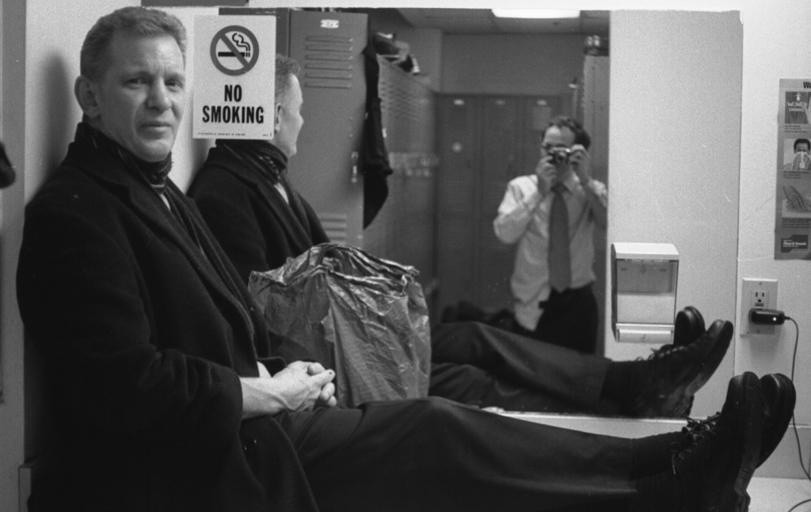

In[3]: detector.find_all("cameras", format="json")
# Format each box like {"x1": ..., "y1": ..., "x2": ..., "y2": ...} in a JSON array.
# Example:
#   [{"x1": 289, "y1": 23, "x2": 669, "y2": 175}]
[{"x1": 542, "y1": 146, "x2": 572, "y2": 168}]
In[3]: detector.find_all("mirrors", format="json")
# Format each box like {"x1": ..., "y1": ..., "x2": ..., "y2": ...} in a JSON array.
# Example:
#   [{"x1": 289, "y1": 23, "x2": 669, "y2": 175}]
[{"x1": 149, "y1": 1, "x2": 747, "y2": 444}]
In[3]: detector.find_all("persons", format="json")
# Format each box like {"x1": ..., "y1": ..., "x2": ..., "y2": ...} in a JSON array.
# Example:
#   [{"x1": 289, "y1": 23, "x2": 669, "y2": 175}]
[
  {"x1": 782, "y1": 141, "x2": 811, "y2": 171},
  {"x1": 185, "y1": 54, "x2": 732, "y2": 418},
  {"x1": 18, "y1": 7, "x2": 796, "y2": 512},
  {"x1": 493, "y1": 115, "x2": 607, "y2": 354}
]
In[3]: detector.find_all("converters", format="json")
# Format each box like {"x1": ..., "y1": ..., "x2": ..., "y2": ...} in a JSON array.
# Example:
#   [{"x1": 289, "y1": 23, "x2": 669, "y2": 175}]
[{"x1": 748, "y1": 306, "x2": 785, "y2": 328}]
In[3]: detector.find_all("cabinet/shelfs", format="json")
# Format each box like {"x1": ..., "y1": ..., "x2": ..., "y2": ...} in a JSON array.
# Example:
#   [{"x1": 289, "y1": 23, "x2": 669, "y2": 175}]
[
  {"x1": 571, "y1": 54, "x2": 605, "y2": 350},
  {"x1": 434, "y1": 93, "x2": 578, "y2": 320},
  {"x1": 287, "y1": 8, "x2": 432, "y2": 350}
]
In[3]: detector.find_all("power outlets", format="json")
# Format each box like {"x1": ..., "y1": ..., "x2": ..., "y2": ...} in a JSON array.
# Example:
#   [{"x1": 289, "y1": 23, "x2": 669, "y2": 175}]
[{"x1": 743, "y1": 279, "x2": 779, "y2": 336}]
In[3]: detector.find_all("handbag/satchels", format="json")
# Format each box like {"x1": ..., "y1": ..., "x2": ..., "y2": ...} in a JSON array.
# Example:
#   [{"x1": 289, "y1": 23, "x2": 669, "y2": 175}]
[{"x1": 251, "y1": 243, "x2": 432, "y2": 406}]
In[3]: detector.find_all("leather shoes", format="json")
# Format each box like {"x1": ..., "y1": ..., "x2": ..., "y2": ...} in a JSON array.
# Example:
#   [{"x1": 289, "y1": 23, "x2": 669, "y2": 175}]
[
  {"x1": 655, "y1": 306, "x2": 733, "y2": 416},
  {"x1": 683, "y1": 371, "x2": 795, "y2": 512}
]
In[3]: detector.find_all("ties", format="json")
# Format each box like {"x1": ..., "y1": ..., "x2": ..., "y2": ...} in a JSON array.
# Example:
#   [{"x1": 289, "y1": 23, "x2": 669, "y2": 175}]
[{"x1": 549, "y1": 184, "x2": 571, "y2": 293}]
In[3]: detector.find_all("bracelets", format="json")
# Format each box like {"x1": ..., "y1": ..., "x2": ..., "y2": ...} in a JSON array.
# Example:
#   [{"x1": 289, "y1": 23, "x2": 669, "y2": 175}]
[{"x1": 580, "y1": 176, "x2": 594, "y2": 187}]
